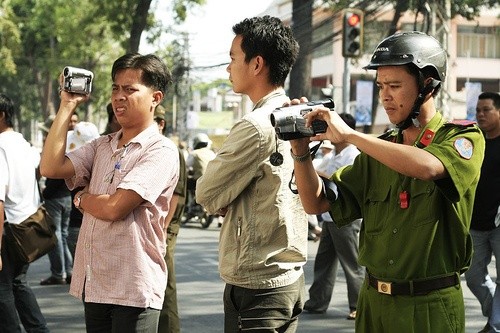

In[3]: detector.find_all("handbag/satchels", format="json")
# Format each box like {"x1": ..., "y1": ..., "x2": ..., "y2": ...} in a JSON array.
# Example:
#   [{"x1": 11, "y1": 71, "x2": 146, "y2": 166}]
[{"x1": 4, "y1": 206, "x2": 59, "y2": 265}]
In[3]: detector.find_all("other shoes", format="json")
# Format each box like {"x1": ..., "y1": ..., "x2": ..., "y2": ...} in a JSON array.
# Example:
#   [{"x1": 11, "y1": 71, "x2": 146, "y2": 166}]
[
  {"x1": 348, "y1": 308, "x2": 356, "y2": 319},
  {"x1": 301, "y1": 307, "x2": 325, "y2": 314},
  {"x1": 40, "y1": 275, "x2": 72, "y2": 285}
]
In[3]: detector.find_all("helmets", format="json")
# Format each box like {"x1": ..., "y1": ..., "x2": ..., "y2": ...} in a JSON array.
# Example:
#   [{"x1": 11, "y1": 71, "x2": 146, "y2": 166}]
[
  {"x1": 362, "y1": 31, "x2": 448, "y2": 82},
  {"x1": 193, "y1": 133, "x2": 210, "y2": 150}
]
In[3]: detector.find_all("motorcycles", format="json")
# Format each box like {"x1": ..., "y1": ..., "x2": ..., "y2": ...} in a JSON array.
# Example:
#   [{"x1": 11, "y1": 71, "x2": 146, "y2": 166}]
[{"x1": 178, "y1": 169, "x2": 219, "y2": 229}]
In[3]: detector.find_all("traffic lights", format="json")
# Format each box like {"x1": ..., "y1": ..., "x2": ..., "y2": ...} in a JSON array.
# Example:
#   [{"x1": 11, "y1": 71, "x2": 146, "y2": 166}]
[{"x1": 342, "y1": 10, "x2": 364, "y2": 59}]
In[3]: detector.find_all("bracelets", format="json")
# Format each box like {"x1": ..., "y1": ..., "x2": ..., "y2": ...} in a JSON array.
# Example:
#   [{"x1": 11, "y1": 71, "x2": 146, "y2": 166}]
[{"x1": 290, "y1": 147, "x2": 311, "y2": 161}]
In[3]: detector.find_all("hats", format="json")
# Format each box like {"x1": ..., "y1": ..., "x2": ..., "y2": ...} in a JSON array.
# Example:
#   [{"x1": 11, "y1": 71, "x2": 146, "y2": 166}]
[
  {"x1": 154, "y1": 105, "x2": 168, "y2": 123},
  {"x1": 107, "y1": 102, "x2": 114, "y2": 114}
]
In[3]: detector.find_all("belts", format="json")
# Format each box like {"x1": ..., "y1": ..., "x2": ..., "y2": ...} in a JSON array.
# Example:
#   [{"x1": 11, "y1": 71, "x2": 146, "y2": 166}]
[{"x1": 365, "y1": 267, "x2": 459, "y2": 298}]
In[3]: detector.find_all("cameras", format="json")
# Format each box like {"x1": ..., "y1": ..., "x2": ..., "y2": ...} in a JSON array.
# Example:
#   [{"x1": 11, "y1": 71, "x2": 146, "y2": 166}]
[
  {"x1": 62, "y1": 66, "x2": 95, "y2": 95},
  {"x1": 271, "y1": 97, "x2": 334, "y2": 140}
]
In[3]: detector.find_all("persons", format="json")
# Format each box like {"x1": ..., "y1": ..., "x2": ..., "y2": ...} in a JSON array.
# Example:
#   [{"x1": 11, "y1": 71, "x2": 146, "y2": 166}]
[
  {"x1": 195, "y1": 15, "x2": 308, "y2": 333},
  {"x1": 154, "y1": 106, "x2": 187, "y2": 333},
  {"x1": 38, "y1": 102, "x2": 122, "y2": 284},
  {"x1": 40, "y1": 52, "x2": 179, "y2": 333},
  {"x1": 0, "y1": 94, "x2": 51, "y2": 333},
  {"x1": 180, "y1": 133, "x2": 216, "y2": 216},
  {"x1": 283, "y1": 31, "x2": 485, "y2": 333},
  {"x1": 465, "y1": 92, "x2": 500, "y2": 333},
  {"x1": 302, "y1": 114, "x2": 366, "y2": 319}
]
